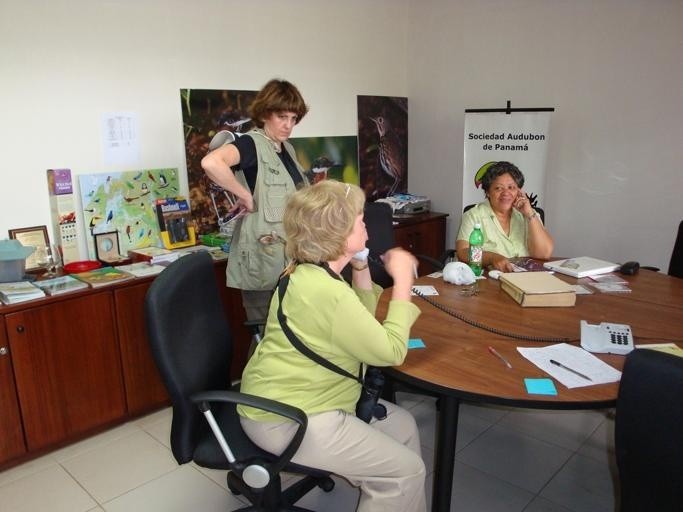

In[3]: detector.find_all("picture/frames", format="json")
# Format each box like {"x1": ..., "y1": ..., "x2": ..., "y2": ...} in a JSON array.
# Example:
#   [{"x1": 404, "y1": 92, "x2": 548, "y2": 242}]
[
  {"x1": 8, "y1": 226, "x2": 55, "y2": 274},
  {"x1": 94, "y1": 230, "x2": 120, "y2": 260}
]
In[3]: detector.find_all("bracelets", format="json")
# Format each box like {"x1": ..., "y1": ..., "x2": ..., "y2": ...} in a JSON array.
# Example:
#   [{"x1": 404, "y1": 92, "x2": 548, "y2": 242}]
[
  {"x1": 527, "y1": 212, "x2": 537, "y2": 221},
  {"x1": 351, "y1": 263, "x2": 369, "y2": 272}
]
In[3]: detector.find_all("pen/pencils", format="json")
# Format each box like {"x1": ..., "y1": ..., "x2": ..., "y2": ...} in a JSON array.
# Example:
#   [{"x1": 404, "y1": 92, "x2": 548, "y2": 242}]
[
  {"x1": 549, "y1": 360, "x2": 596, "y2": 382},
  {"x1": 488, "y1": 345, "x2": 514, "y2": 370}
]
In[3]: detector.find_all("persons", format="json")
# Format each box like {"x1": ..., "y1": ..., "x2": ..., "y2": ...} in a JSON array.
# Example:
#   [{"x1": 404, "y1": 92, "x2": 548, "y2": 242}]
[
  {"x1": 456, "y1": 161, "x2": 554, "y2": 270},
  {"x1": 236, "y1": 179, "x2": 427, "y2": 512},
  {"x1": 201, "y1": 80, "x2": 309, "y2": 356}
]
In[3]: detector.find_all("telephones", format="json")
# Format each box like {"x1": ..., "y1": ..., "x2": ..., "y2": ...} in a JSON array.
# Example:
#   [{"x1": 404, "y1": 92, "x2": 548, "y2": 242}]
[{"x1": 353, "y1": 246, "x2": 369, "y2": 261}]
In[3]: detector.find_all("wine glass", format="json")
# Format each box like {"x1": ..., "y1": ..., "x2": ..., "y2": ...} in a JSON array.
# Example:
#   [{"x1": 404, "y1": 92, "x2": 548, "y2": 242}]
[{"x1": 37, "y1": 244, "x2": 59, "y2": 278}]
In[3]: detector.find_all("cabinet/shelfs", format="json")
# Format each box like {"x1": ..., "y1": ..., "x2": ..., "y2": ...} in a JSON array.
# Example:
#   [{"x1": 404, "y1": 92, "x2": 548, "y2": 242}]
[
  {"x1": 0, "y1": 307, "x2": 26, "y2": 471},
  {"x1": 396, "y1": 214, "x2": 447, "y2": 276},
  {"x1": 114, "y1": 259, "x2": 253, "y2": 421},
  {"x1": 5, "y1": 285, "x2": 129, "y2": 461}
]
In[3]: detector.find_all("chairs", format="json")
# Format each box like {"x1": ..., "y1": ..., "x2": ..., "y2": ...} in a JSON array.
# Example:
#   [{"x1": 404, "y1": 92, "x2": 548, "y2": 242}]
[
  {"x1": 144, "y1": 251, "x2": 335, "y2": 511},
  {"x1": 341, "y1": 202, "x2": 445, "y2": 410},
  {"x1": 638, "y1": 220, "x2": 683, "y2": 280},
  {"x1": 441, "y1": 204, "x2": 544, "y2": 273},
  {"x1": 614, "y1": 348, "x2": 683, "y2": 511}
]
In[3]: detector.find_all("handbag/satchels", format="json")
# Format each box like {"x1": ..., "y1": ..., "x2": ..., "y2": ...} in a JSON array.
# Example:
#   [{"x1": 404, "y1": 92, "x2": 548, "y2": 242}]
[{"x1": 355, "y1": 366, "x2": 387, "y2": 424}]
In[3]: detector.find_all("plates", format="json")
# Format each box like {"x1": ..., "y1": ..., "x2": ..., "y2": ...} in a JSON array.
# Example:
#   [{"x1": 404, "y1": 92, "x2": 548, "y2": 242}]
[{"x1": 64, "y1": 261, "x2": 101, "y2": 273}]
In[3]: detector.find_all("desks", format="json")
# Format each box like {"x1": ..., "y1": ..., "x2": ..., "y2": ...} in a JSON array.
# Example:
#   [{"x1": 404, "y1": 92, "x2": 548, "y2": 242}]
[{"x1": 375, "y1": 256, "x2": 683, "y2": 511}]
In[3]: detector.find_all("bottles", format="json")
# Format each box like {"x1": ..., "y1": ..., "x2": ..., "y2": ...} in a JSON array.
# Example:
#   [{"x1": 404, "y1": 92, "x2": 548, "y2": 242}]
[{"x1": 469, "y1": 224, "x2": 484, "y2": 276}]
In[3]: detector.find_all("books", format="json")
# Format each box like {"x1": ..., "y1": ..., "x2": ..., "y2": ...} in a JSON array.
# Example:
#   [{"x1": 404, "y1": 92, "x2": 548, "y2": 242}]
[
  {"x1": 0, "y1": 281, "x2": 46, "y2": 305},
  {"x1": 128, "y1": 247, "x2": 179, "y2": 263},
  {"x1": 70, "y1": 266, "x2": 135, "y2": 288},
  {"x1": 543, "y1": 255, "x2": 621, "y2": 278},
  {"x1": 497, "y1": 272, "x2": 576, "y2": 308},
  {"x1": 32, "y1": 275, "x2": 88, "y2": 296},
  {"x1": 114, "y1": 261, "x2": 167, "y2": 278}
]
